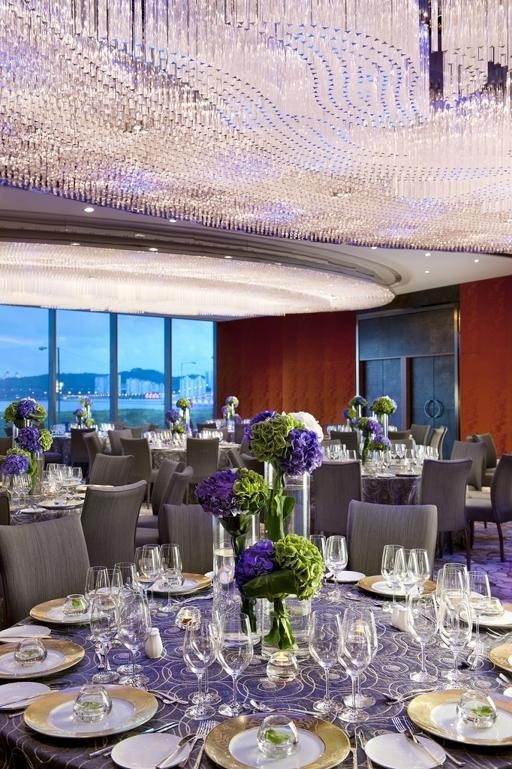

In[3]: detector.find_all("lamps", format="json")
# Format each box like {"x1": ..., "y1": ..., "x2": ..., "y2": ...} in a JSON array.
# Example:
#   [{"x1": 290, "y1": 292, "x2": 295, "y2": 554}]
[{"x1": 1, "y1": 238, "x2": 398, "y2": 322}]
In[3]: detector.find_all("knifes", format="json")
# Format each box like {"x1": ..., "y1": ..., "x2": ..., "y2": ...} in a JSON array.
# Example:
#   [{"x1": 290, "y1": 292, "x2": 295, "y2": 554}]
[
  {"x1": 358, "y1": 729, "x2": 375, "y2": 769},
  {"x1": 0, "y1": 690, "x2": 58, "y2": 709},
  {"x1": 349, "y1": 728, "x2": 358, "y2": 769},
  {"x1": 0, "y1": 634, "x2": 52, "y2": 640},
  {"x1": 155, "y1": 733, "x2": 197, "y2": 769}
]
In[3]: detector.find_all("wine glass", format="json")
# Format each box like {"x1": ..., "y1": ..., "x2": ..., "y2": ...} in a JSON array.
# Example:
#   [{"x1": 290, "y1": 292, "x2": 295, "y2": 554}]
[
  {"x1": 51, "y1": 422, "x2": 115, "y2": 438},
  {"x1": 0, "y1": 464, "x2": 83, "y2": 517},
  {"x1": 84, "y1": 534, "x2": 492, "y2": 722},
  {"x1": 321, "y1": 441, "x2": 439, "y2": 478},
  {"x1": 206, "y1": 418, "x2": 251, "y2": 430},
  {"x1": 326, "y1": 424, "x2": 398, "y2": 441},
  {"x1": 142, "y1": 429, "x2": 224, "y2": 449}
]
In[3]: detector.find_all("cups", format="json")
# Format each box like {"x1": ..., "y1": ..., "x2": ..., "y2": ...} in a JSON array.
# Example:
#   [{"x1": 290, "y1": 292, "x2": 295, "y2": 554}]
[
  {"x1": 253, "y1": 715, "x2": 300, "y2": 758},
  {"x1": 481, "y1": 594, "x2": 504, "y2": 618},
  {"x1": 456, "y1": 688, "x2": 497, "y2": 730},
  {"x1": 15, "y1": 637, "x2": 47, "y2": 668},
  {"x1": 61, "y1": 595, "x2": 88, "y2": 620},
  {"x1": 73, "y1": 686, "x2": 112, "y2": 725}
]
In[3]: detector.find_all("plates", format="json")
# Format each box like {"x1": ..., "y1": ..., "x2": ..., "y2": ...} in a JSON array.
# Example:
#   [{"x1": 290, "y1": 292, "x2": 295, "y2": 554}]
[
  {"x1": 131, "y1": 572, "x2": 212, "y2": 595},
  {"x1": 204, "y1": 711, "x2": 351, "y2": 769},
  {"x1": 0, "y1": 640, "x2": 85, "y2": 680},
  {"x1": 461, "y1": 605, "x2": 512, "y2": 628},
  {"x1": 109, "y1": 733, "x2": 190, "y2": 767},
  {"x1": 488, "y1": 640, "x2": 512, "y2": 673},
  {"x1": 30, "y1": 594, "x2": 114, "y2": 623},
  {"x1": 359, "y1": 574, "x2": 437, "y2": 597},
  {"x1": 1, "y1": 683, "x2": 52, "y2": 709},
  {"x1": 327, "y1": 570, "x2": 367, "y2": 582},
  {"x1": 0, "y1": 571, "x2": 512, "y2": 769},
  {"x1": 75, "y1": 485, "x2": 114, "y2": 491},
  {"x1": 404, "y1": 689, "x2": 512, "y2": 745},
  {"x1": 1, "y1": 625, "x2": 52, "y2": 643},
  {"x1": 26, "y1": 683, "x2": 159, "y2": 737}
]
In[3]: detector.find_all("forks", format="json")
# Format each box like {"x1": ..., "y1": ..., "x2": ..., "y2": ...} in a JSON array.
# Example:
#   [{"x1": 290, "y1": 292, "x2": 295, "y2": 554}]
[
  {"x1": 177, "y1": 719, "x2": 208, "y2": 768},
  {"x1": 402, "y1": 714, "x2": 466, "y2": 767},
  {"x1": 390, "y1": 716, "x2": 447, "y2": 769},
  {"x1": 191, "y1": 720, "x2": 216, "y2": 769}
]
